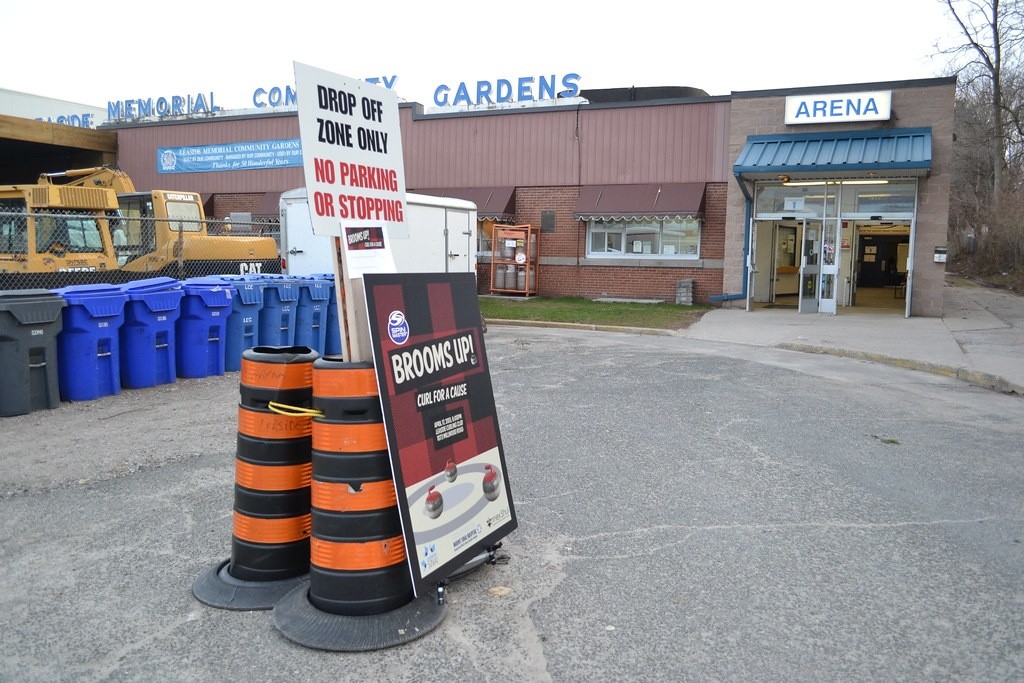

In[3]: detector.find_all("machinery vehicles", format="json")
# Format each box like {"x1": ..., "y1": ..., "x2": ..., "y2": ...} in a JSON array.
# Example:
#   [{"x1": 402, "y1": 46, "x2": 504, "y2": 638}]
[{"x1": 0, "y1": 162, "x2": 281, "y2": 290}]
